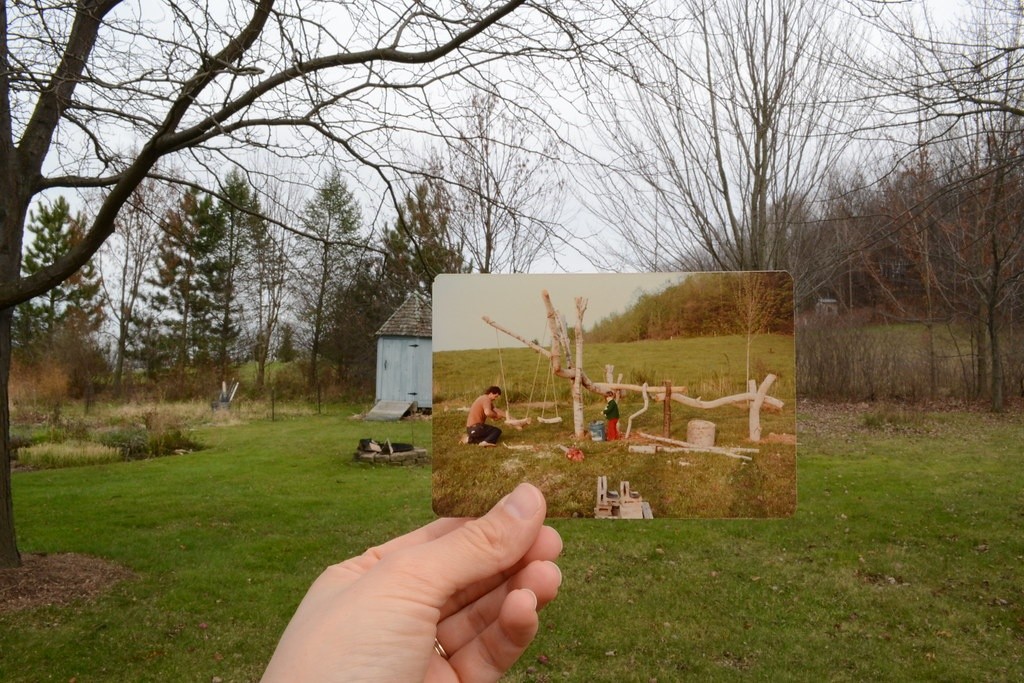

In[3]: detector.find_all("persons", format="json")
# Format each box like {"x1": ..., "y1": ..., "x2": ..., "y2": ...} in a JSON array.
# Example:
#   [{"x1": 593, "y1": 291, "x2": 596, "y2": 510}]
[
  {"x1": 256, "y1": 483, "x2": 563, "y2": 683},
  {"x1": 458, "y1": 386, "x2": 503, "y2": 447},
  {"x1": 600, "y1": 391, "x2": 620, "y2": 441}
]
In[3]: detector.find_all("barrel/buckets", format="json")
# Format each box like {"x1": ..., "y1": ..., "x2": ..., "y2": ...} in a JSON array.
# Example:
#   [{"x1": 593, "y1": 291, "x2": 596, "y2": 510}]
[{"x1": 589, "y1": 421, "x2": 604, "y2": 441}]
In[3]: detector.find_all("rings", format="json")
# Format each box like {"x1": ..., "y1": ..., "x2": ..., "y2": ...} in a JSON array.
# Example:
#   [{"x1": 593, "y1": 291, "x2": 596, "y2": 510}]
[{"x1": 434, "y1": 639, "x2": 449, "y2": 659}]
